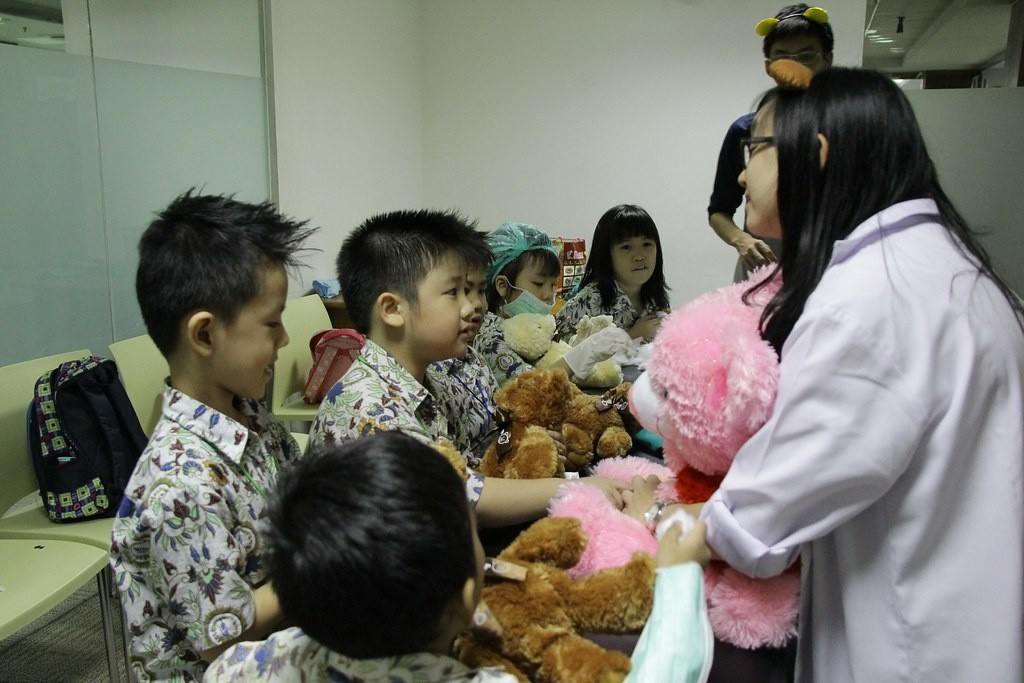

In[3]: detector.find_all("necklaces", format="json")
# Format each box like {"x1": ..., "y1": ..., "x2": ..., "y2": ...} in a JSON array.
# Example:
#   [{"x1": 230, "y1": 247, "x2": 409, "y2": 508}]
[
  {"x1": 359, "y1": 355, "x2": 433, "y2": 440},
  {"x1": 448, "y1": 369, "x2": 488, "y2": 408},
  {"x1": 241, "y1": 445, "x2": 284, "y2": 499}
]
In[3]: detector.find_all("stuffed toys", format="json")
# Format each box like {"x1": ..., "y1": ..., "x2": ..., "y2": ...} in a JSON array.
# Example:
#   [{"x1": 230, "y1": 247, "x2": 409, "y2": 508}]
[{"x1": 426, "y1": 261, "x2": 802, "y2": 683}]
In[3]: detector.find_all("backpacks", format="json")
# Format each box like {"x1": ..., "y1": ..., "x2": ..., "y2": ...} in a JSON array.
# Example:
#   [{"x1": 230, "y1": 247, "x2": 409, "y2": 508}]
[
  {"x1": 27, "y1": 356, "x2": 150, "y2": 525},
  {"x1": 304, "y1": 329, "x2": 366, "y2": 404}
]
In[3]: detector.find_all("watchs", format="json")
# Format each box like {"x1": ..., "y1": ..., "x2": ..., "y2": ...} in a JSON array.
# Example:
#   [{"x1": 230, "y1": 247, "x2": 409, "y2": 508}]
[{"x1": 644, "y1": 501, "x2": 667, "y2": 521}]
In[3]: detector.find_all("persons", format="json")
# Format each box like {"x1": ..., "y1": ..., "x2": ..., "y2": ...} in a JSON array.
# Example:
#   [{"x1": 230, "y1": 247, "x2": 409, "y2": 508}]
[
  {"x1": 109, "y1": 194, "x2": 715, "y2": 683},
  {"x1": 621, "y1": 67, "x2": 1024, "y2": 683},
  {"x1": 707, "y1": 4, "x2": 834, "y2": 283}
]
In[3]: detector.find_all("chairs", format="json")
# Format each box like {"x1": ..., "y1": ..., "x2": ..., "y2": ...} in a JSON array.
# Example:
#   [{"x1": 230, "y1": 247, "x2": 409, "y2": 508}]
[{"x1": 0, "y1": 293, "x2": 332, "y2": 683}]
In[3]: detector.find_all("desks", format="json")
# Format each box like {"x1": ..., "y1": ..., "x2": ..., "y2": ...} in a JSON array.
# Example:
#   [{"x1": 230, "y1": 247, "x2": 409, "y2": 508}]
[{"x1": 301, "y1": 282, "x2": 354, "y2": 328}]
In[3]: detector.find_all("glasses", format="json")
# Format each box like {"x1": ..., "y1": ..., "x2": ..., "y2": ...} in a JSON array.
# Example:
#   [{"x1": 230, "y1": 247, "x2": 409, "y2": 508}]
[{"x1": 740, "y1": 136, "x2": 773, "y2": 170}]
